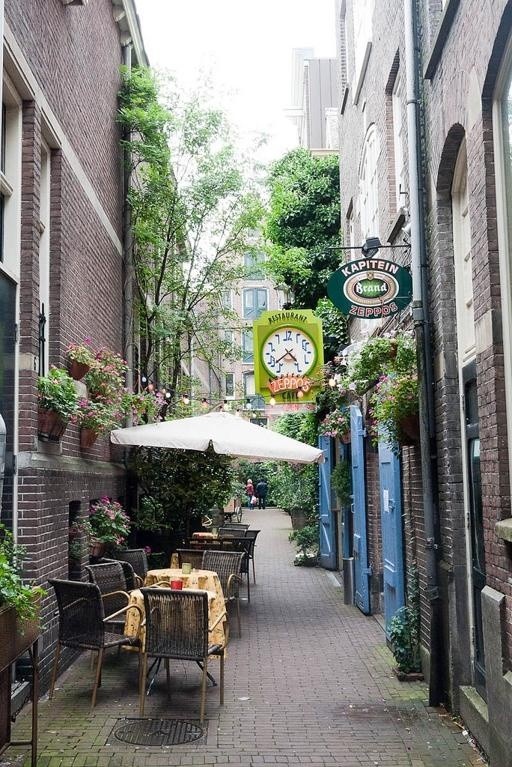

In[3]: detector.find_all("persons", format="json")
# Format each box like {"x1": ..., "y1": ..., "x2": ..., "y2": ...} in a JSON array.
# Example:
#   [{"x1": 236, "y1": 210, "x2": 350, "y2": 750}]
[
  {"x1": 246, "y1": 478, "x2": 256, "y2": 510},
  {"x1": 255, "y1": 478, "x2": 268, "y2": 510}
]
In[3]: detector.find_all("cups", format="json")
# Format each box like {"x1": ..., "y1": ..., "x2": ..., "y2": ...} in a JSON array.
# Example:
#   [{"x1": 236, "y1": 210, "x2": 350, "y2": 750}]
[
  {"x1": 182, "y1": 563, "x2": 192, "y2": 574},
  {"x1": 171, "y1": 580, "x2": 182, "y2": 591},
  {"x1": 212, "y1": 528, "x2": 218, "y2": 535}
]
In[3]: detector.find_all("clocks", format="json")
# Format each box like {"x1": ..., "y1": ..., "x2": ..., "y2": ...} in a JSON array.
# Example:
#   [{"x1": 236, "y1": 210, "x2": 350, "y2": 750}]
[{"x1": 252, "y1": 309, "x2": 325, "y2": 404}]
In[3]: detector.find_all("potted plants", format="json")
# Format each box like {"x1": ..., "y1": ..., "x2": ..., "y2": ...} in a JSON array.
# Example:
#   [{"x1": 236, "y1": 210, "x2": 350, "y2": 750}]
[{"x1": 263, "y1": 461, "x2": 319, "y2": 532}]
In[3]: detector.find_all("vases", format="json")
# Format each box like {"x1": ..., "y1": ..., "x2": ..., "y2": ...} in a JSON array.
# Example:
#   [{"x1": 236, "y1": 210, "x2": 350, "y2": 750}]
[
  {"x1": 339, "y1": 429, "x2": 351, "y2": 445},
  {"x1": 38, "y1": 407, "x2": 69, "y2": 443},
  {"x1": 69, "y1": 359, "x2": 90, "y2": 380},
  {"x1": 79, "y1": 427, "x2": 98, "y2": 450},
  {"x1": 399, "y1": 415, "x2": 419, "y2": 442}
]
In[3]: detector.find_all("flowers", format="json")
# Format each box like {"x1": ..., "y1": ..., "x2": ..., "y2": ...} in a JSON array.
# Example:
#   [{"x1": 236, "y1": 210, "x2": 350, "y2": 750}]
[
  {"x1": 90, "y1": 497, "x2": 131, "y2": 556},
  {"x1": 65, "y1": 338, "x2": 100, "y2": 370},
  {"x1": 369, "y1": 374, "x2": 419, "y2": 458},
  {"x1": 80, "y1": 349, "x2": 168, "y2": 436},
  {"x1": 318, "y1": 408, "x2": 350, "y2": 439},
  {"x1": 37, "y1": 364, "x2": 80, "y2": 424}
]
